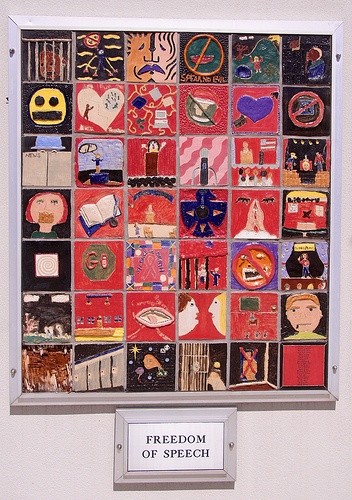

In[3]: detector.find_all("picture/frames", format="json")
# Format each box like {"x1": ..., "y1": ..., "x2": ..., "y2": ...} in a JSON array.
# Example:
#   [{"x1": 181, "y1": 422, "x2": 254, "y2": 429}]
[{"x1": 5, "y1": 14, "x2": 343, "y2": 407}]
